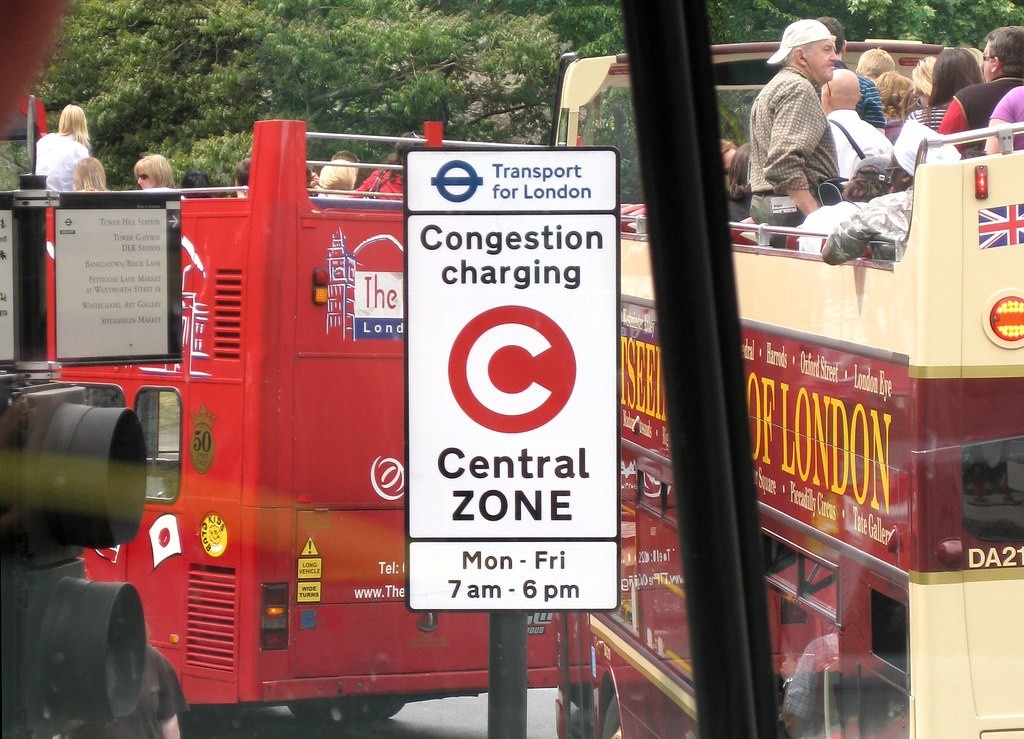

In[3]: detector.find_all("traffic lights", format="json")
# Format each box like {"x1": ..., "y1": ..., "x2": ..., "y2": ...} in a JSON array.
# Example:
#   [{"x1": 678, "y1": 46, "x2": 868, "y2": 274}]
[{"x1": 2, "y1": 374, "x2": 146, "y2": 739}]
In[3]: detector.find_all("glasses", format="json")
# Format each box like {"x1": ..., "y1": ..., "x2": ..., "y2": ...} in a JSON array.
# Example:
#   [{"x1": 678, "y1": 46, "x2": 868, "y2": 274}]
[
  {"x1": 982, "y1": 53, "x2": 998, "y2": 61},
  {"x1": 136, "y1": 174, "x2": 148, "y2": 180}
]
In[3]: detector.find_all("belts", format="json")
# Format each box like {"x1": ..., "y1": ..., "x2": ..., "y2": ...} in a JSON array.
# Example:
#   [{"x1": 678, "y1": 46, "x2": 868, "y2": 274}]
[{"x1": 752, "y1": 191, "x2": 786, "y2": 197}]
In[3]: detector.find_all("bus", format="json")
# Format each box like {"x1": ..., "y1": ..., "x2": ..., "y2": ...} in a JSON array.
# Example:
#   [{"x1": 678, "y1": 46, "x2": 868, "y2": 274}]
[
  {"x1": 547, "y1": 37, "x2": 1024, "y2": 739},
  {"x1": 2, "y1": 92, "x2": 602, "y2": 727}
]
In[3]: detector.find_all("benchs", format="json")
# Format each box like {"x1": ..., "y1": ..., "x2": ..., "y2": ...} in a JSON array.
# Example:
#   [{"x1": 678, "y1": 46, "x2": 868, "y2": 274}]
[{"x1": 621, "y1": 202, "x2": 872, "y2": 258}]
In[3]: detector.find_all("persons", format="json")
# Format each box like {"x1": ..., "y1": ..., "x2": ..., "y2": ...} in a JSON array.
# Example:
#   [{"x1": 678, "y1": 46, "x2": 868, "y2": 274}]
[
  {"x1": 307, "y1": 132, "x2": 424, "y2": 200},
  {"x1": 720, "y1": 16, "x2": 1024, "y2": 266},
  {"x1": 235, "y1": 158, "x2": 251, "y2": 198},
  {"x1": 36, "y1": 105, "x2": 107, "y2": 191},
  {"x1": 133, "y1": 154, "x2": 210, "y2": 190}
]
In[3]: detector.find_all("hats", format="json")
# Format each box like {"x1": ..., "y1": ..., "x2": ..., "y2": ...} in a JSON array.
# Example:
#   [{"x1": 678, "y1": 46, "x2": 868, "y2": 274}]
[
  {"x1": 767, "y1": 19, "x2": 834, "y2": 65},
  {"x1": 852, "y1": 156, "x2": 891, "y2": 181}
]
